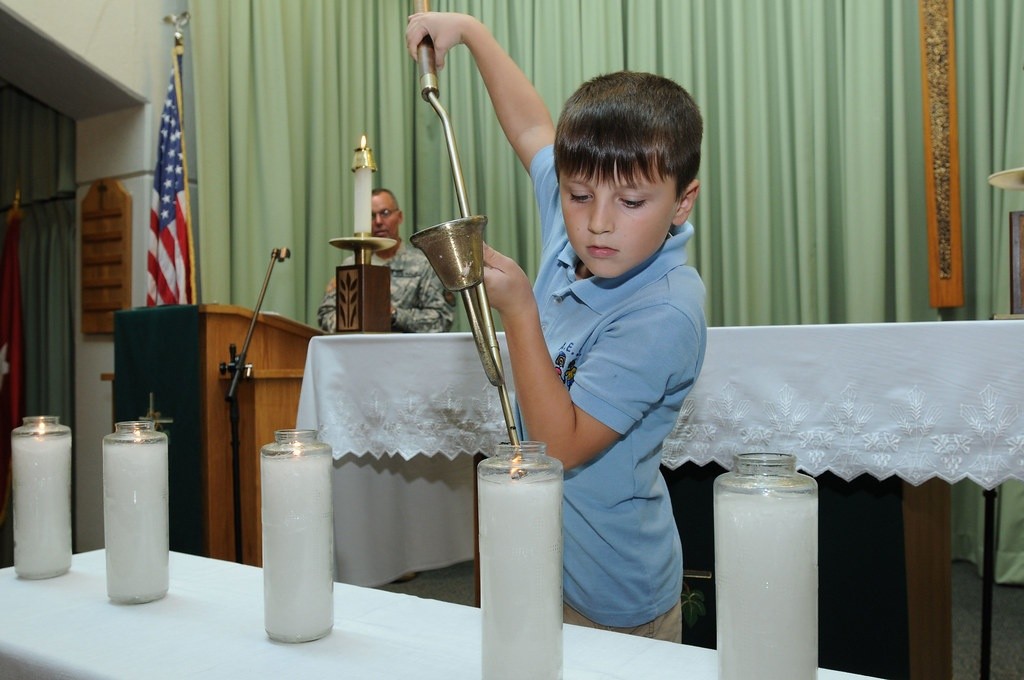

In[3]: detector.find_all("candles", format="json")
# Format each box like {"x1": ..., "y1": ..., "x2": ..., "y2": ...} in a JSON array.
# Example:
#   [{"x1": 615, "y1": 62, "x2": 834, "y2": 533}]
[
  {"x1": 714, "y1": 481, "x2": 818, "y2": 680},
  {"x1": 259, "y1": 453, "x2": 336, "y2": 644},
  {"x1": 11, "y1": 422, "x2": 73, "y2": 579},
  {"x1": 105, "y1": 432, "x2": 170, "y2": 604},
  {"x1": 481, "y1": 472, "x2": 562, "y2": 678},
  {"x1": 351, "y1": 134, "x2": 376, "y2": 237}
]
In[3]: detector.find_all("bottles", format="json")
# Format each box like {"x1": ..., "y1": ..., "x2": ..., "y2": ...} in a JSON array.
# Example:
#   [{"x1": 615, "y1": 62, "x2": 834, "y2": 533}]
[
  {"x1": 259, "y1": 428, "x2": 336, "y2": 645},
  {"x1": 711, "y1": 454, "x2": 819, "y2": 680},
  {"x1": 11, "y1": 416, "x2": 73, "y2": 581},
  {"x1": 475, "y1": 442, "x2": 562, "y2": 680},
  {"x1": 101, "y1": 418, "x2": 167, "y2": 603}
]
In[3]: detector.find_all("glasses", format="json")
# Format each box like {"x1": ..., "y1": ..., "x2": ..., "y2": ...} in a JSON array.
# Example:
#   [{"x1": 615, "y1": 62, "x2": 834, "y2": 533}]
[{"x1": 372, "y1": 208, "x2": 400, "y2": 219}]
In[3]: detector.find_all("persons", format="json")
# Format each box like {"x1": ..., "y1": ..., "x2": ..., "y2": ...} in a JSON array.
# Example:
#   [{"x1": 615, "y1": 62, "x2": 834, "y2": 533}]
[
  {"x1": 318, "y1": 187, "x2": 457, "y2": 334},
  {"x1": 405, "y1": 12, "x2": 708, "y2": 644}
]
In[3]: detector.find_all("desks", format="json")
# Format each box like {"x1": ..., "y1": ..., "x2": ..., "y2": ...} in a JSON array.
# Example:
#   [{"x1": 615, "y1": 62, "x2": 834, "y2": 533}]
[{"x1": 310, "y1": 320, "x2": 1024, "y2": 680}]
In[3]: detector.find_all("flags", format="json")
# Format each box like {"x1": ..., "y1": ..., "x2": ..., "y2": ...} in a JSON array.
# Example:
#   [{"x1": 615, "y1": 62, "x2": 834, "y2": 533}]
[
  {"x1": 0, "y1": 200, "x2": 20, "y2": 524},
  {"x1": 147, "y1": 49, "x2": 199, "y2": 309}
]
